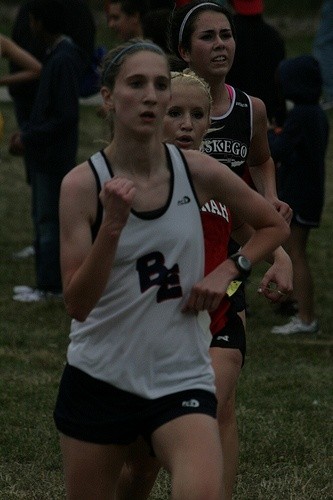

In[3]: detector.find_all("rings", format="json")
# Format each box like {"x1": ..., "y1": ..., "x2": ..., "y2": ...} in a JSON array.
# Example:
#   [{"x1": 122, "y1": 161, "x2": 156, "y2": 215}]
[{"x1": 276, "y1": 290, "x2": 283, "y2": 297}]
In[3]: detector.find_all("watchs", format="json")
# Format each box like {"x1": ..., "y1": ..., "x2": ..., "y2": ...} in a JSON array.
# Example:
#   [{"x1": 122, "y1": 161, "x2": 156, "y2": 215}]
[{"x1": 231, "y1": 254, "x2": 252, "y2": 280}]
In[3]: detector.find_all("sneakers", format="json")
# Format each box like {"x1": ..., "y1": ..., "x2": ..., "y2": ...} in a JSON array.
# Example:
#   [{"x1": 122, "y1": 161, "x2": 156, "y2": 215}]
[
  {"x1": 13, "y1": 288, "x2": 64, "y2": 302},
  {"x1": 14, "y1": 245, "x2": 35, "y2": 259},
  {"x1": 12, "y1": 285, "x2": 34, "y2": 294},
  {"x1": 272, "y1": 315, "x2": 318, "y2": 335}
]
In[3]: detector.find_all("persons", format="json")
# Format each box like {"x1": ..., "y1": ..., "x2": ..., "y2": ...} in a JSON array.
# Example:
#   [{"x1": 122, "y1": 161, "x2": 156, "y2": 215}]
[
  {"x1": 170, "y1": 2, "x2": 293, "y2": 499},
  {"x1": 115, "y1": 68, "x2": 293, "y2": 500},
  {"x1": 0, "y1": 0, "x2": 333, "y2": 334},
  {"x1": 53, "y1": 39, "x2": 290, "y2": 500}
]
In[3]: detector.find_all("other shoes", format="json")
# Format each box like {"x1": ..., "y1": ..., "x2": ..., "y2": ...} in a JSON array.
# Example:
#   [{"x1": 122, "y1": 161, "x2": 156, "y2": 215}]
[{"x1": 278, "y1": 299, "x2": 300, "y2": 317}]
